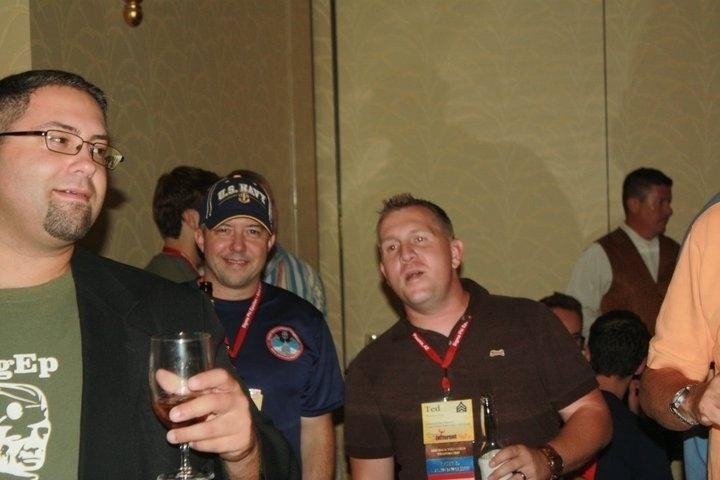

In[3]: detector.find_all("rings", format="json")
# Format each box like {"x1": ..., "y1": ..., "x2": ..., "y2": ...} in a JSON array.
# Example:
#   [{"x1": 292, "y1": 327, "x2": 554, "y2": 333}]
[{"x1": 515, "y1": 471, "x2": 526, "y2": 479}]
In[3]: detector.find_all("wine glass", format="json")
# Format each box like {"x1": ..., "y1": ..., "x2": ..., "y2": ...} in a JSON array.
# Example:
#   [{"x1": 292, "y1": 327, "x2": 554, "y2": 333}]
[{"x1": 146, "y1": 331, "x2": 214, "y2": 480}]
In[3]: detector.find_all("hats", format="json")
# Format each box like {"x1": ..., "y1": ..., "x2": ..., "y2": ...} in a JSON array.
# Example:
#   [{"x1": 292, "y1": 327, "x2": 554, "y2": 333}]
[{"x1": 200, "y1": 176, "x2": 273, "y2": 236}]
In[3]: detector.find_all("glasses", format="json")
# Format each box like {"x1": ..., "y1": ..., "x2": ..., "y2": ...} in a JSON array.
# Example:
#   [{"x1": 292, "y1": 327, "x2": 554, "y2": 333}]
[{"x1": 0, "y1": 130, "x2": 124, "y2": 170}]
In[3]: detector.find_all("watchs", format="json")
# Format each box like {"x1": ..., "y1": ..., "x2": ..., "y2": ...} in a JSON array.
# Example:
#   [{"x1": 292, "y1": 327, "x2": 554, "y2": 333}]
[
  {"x1": 670, "y1": 377, "x2": 699, "y2": 428},
  {"x1": 538, "y1": 441, "x2": 565, "y2": 478}
]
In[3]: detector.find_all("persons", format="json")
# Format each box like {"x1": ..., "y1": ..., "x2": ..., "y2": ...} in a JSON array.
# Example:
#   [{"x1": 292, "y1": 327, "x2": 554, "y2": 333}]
[
  {"x1": 0, "y1": 69, "x2": 302, "y2": 479},
  {"x1": 343, "y1": 193, "x2": 617, "y2": 480},
  {"x1": 570, "y1": 166, "x2": 683, "y2": 343},
  {"x1": 539, "y1": 289, "x2": 585, "y2": 354},
  {"x1": 144, "y1": 166, "x2": 220, "y2": 284},
  {"x1": 235, "y1": 169, "x2": 328, "y2": 320},
  {"x1": 190, "y1": 168, "x2": 345, "y2": 479},
  {"x1": 639, "y1": 189, "x2": 720, "y2": 479},
  {"x1": 588, "y1": 309, "x2": 675, "y2": 478}
]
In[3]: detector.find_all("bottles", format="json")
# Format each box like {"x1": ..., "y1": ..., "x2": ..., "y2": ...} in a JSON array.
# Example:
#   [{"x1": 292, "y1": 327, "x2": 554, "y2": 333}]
[{"x1": 472, "y1": 394, "x2": 513, "y2": 480}]
